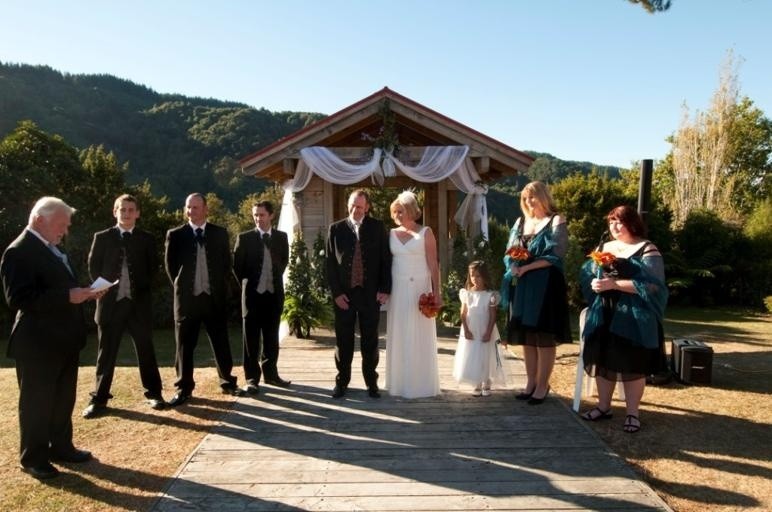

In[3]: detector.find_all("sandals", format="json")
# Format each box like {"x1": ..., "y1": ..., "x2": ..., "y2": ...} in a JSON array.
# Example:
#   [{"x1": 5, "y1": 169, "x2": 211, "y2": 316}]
[
  {"x1": 623, "y1": 415, "x2": 641, "y2": 432},
  {"x1": 579, "y1": 407, "x2": 613, "y2": 420}
]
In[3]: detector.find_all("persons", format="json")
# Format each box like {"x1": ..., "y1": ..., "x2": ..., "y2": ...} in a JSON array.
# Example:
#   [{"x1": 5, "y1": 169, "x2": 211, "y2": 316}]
[
  {"x1": 580, "y1": 205, "x2": 669, "y2": 433},
  {"x1": 456, "y1": 261, "x2": 501, "y2": 398},
  {"x1": 377, "y1": 190, "x2": 441, "y2": 400},
  {"x1": 5, "y1": 196, "x2": 110, "y2": 480},
  {"x1": 497, "y1": 181, "x2": 569, "y2": 404},
  {"x1": 83, "y1": 193, "x2": 166, "y2": 418},
  {"x1": 164, "y1": 192, "x2": 246, "y2": 407},
  {"x1": 326, "y1": 190, "x2": 390, "y2": 398},
  {"x1": 233, "y1": 201, "x2": 291, "y2": 393}
]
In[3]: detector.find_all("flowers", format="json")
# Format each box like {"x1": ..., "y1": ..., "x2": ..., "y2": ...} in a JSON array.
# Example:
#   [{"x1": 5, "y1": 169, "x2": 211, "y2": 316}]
[
  {"x1": 418, "y1": 292, "x2": 461, "y2": 318},
  {"x1": 586, "y1": 249, "x2": 616, "y2": 279},
  {"x1": 361, "y1": 127, "x2": 403, "y2": 157},
  {"x1": 505, "y1": 246, "x2": 532, "y2": 287}
]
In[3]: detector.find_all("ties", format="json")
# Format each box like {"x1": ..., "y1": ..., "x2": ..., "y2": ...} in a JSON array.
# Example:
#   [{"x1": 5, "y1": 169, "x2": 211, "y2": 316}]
[
  {"x1": 263, "y1": 233, "x2": 269, "y2": 246},
  {"x1": 196, "y1": 228, "x2": 203, "y2": 244},
  {"x1": 122, "y1": 232, "x2": 131, "y2": 243}
]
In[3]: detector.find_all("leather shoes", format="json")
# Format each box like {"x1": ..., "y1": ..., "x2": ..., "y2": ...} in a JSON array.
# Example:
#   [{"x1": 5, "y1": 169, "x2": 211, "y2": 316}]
[
  {"x1": 169, "y1": 393, "x2": 191, "y2": 406},
  {"x1": 331, "y1": 379, "x2": 346, "y2": 397},
  {"x1": 54, "y1": 450, "x2": 91, "y2": 463},
  {"x1": 82, "y1": 404, "x2": 107, "y2": 418},
  {"x1": 21, "y1": 462, "x2": 58, "y2": 478},
  {"x1": 472, "y1": 387, "x2": 490, "y2": 397},
  {"x1": 369, "y1": 386, "x2": 380, "y2": 398},
  {"x1": 222, "y1": 377, "x2": 293, "y2": 396},
  {"x1": 149, "y1": 398, "x2": 164, "y2": 409}
]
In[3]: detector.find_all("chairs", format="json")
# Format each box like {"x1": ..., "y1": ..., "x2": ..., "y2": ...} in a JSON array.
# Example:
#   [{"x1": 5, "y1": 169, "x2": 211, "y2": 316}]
[{"x1": 573, "y1": 307, "x2": 626, "y2": 413}]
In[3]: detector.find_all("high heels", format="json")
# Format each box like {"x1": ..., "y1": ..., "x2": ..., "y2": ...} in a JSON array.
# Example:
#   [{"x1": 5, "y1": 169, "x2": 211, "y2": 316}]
[
  {"x1": 516, "y1": 385, "x2": 536, "y2": 399},
  {"x1": 528, "y1": 385, "x2": 550, "y2": 403}
]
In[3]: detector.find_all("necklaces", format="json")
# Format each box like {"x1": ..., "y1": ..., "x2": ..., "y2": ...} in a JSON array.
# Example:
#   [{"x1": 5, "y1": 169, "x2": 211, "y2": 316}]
[
  {"x1": 615, "y1": 246, "x2": 627, "y2": 253},
  {"x1": 532, "y1": 221, "x2": 542, "y2": 227}
]
